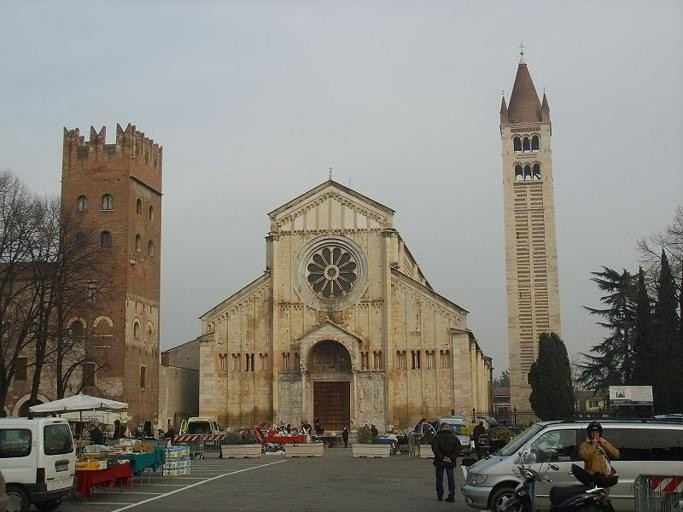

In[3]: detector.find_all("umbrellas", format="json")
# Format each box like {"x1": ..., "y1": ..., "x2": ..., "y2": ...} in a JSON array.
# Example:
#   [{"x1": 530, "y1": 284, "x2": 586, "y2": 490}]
[
  {"x1": 51, "y1": 410, "x2": 131, "y2": 433},
  {"x1": 27, "y1": 390, "x2": 127, "y2": 451}
]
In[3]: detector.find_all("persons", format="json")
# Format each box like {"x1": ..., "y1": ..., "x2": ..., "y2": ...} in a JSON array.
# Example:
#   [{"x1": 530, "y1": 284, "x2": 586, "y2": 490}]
[
  {"x1": 579, "y1": 421, "x2": 620, "y2": 496},
  {"x1": 111, "y1": 420, "x2": 123, "y2": 439},
  {"x1": 340, "y1": 426, "x2": 348, "y2": 447},
  {"x1": 431, "y1": 421, "x2": 460, "y2": 502},
  {"x1": 89, "y1": 424, "x2": 103, "y2": 444},
  {"x1": 286, "y1": 424, "x2": 291, "y2": 433},
  {"x1": 472, "y1": 421, "x2": 485, "y2": 457},
  {"x1": 301, "y1": 420, "x2": 312, "y2": 434},
  {"x1": 314, "y1": 418, "x2": 324, "y2": 434}
]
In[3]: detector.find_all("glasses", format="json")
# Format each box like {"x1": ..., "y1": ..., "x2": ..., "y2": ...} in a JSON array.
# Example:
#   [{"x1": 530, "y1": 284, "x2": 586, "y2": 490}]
[{"x1": 590, "y1": 429, "x2": 599, "y2": 433}]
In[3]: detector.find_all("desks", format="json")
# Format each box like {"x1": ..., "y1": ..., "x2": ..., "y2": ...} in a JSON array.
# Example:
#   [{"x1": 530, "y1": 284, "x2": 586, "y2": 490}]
[
  {"x1": 71, "y1": 460, "x2": 132, "y2": 505},
  {"x1": 264, "y1": 432, "x2": 342, "y2": 454},
  {"x1": 110, "y1": 446, "x2": 162, "y2": 485}
]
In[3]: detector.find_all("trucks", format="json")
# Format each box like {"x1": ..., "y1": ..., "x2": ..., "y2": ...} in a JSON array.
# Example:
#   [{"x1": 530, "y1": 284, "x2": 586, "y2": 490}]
[{"x1": 260, "y1": 429, "x2": 304, "y2": 444}]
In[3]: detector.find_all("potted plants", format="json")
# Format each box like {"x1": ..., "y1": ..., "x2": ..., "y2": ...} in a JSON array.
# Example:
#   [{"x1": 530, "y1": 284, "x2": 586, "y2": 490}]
[
  {"x1": 219, "y1": 432, "x2": 263, "y2": 459},
  {"x1": 412, "y1": 431, "x2": 438, "y2": 460},
  {"x1": 353, "y1": 426, "x2": 393, "y2": 460}
]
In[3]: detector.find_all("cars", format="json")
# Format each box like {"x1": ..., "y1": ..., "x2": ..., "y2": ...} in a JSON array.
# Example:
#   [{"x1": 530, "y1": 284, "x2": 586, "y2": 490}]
[
  {"x1": 375, "y1": 430, "x2": 421, "y2": 446},
  {"x1": 235, "y1": 422, "x2": 269, "y2": 442}
]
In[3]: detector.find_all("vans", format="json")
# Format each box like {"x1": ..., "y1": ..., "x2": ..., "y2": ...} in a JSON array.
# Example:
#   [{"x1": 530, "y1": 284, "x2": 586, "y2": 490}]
[
  {"x1": 460, "y1": 413, "x2": 683, "y2": 511},
  {"x1": 475, "y1": 416, "x2": 528, "y2": 456},
  {"x1": 178, "y1": 416, "x2": 222, "y2": 445},
  {"x1": 0, "y1": 415, "x2": 77, "y2": 511},
  {"x1": 419, "y1": 414, "x2": 476, "y2": 457}
]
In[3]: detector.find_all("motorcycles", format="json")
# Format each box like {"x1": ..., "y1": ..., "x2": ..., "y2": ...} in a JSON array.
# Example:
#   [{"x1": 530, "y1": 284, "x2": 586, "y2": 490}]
[{"x1": 503, "y1": 444, "x2": 617, "y2": 511}]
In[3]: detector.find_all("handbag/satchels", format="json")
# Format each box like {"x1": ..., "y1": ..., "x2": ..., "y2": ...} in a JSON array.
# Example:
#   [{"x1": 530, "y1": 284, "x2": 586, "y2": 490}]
[{"x1": 609, "y1": 466, "x2": 620, "y2": 485}]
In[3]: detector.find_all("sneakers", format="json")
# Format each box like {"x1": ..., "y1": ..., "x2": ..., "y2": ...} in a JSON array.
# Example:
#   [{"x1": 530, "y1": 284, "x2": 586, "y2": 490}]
[
  {"x1": 437, "y1": 495, "x2": 443, "y2": 501},
  {"x1": 444, "y1": 495, "x2": 455, "y2": 503}
]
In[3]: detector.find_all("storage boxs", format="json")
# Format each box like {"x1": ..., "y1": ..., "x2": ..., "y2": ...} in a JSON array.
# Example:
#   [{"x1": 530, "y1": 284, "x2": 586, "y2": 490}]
[{"x1": 143, "y1": 445, "x2": 191, "y2": 477}]
